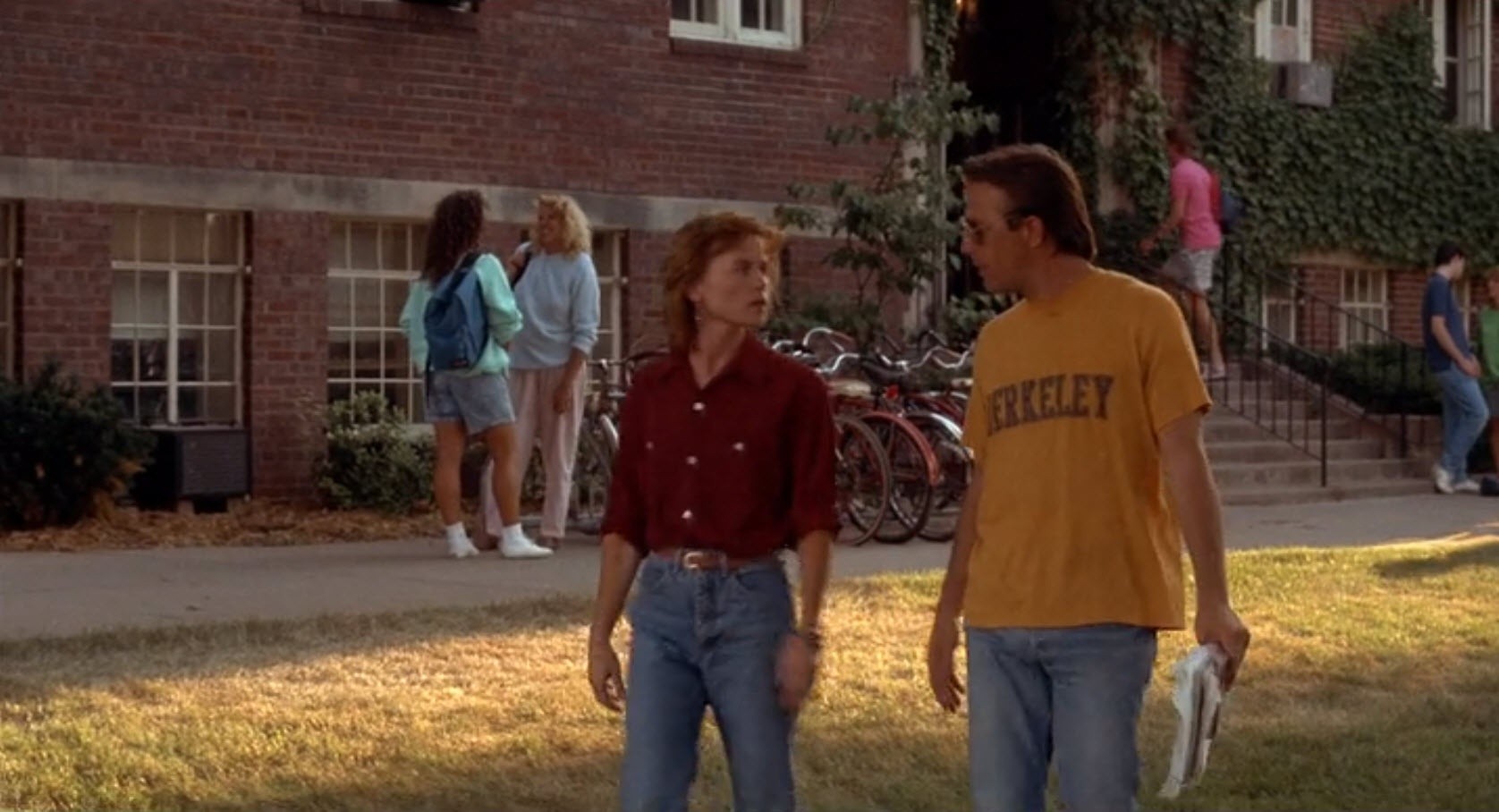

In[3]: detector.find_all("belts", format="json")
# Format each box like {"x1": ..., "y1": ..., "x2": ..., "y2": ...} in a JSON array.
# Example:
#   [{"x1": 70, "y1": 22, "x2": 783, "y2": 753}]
[{"x1": 660, "y1": 547, "x2": 776, "y2": 574}]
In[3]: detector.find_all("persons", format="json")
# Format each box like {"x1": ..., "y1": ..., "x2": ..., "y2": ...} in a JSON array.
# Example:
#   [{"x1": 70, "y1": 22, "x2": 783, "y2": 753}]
[
  {"x1": 926, "y1": 142, "x2": 1251, "y2": 812},
  {"x1": 587, "y1": 211, "x2": 841, "y2": 812},
  {"x1": 475, "y1": 193, "x2": 602, "y2": 550},
  {"x1": 399, "y1": 188, "x2": 552, "y2": 556},
  {"x1": 1140, "y1": 125, "x2": 1227, "y2": 383},
  {"x1": 1478, "y1": 267, "x2": 1499, "y2": 476},
  {"x1": 1423, "y1": 239, "x2": 1490, "y2": 493}
]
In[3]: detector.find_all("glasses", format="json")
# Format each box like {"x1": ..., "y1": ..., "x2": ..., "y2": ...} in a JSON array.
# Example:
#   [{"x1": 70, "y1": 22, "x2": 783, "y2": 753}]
[{"x1": 956, "y1": 214, "x2": 1029, "y2": 246}]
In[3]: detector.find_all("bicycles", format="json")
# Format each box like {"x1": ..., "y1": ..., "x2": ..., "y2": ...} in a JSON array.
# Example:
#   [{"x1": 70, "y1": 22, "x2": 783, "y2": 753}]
[
  {"x1": 573, "y1": 355, "x2": 649, "y2": 534},
  {"x1": 766, "y1": 330, "x2": 984, "y2": 550}
]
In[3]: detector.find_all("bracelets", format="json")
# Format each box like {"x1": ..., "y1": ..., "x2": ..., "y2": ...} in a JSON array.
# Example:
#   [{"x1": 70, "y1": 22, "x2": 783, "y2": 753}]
[{"x1": 790, "y1": 629, "x2": 824, "y2": 648}]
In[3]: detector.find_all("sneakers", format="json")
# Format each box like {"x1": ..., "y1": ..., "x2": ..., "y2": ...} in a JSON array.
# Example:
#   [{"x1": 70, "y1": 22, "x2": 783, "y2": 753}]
[
  {"x1": 445, "y1": 522, "x2": 481, "y2": 559},
  {"x1": 1457, "y1": 480, "x2": 1480, "y2": 492},
  {"x1": 501, "y1": 523, "x2": 554, "y2": 557},
  {"x1": 1431, "y1": 465, "x2": 1454, "y2": 494}
]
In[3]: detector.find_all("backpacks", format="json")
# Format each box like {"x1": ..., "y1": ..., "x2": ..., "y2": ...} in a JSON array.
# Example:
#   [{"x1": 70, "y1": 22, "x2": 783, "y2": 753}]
[
  {"x1": 425, "y1": 253, "x2": 488, "y2": 371},
  {"x1": 1193, "y1": 153, "x2": 1244, "y2": 236}
]
